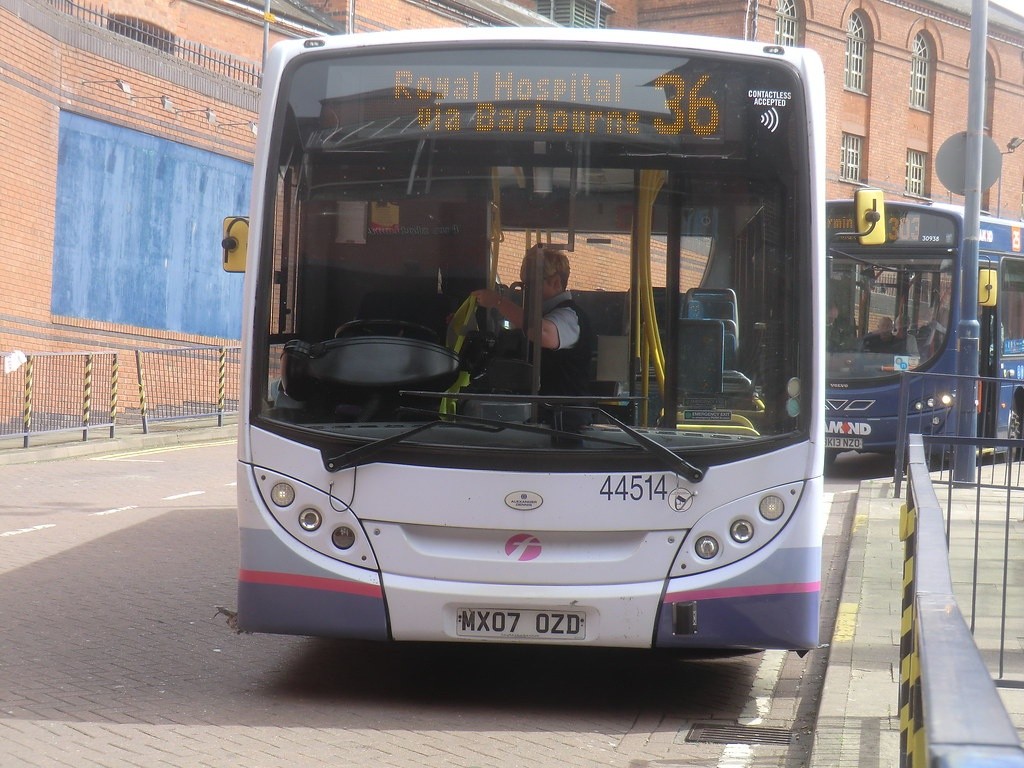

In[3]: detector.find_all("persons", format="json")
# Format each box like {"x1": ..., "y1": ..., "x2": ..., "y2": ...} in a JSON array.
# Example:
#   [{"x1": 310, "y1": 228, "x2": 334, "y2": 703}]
[
  {"x1": 861, "y1": 315, "x2": 920, "y2": 368},
  {"x1": 446, "y1": 245, "x2": 597, "y2": 429},
  {"x1": 826, "y1": 300, "x2": 857, "y2": 350}
]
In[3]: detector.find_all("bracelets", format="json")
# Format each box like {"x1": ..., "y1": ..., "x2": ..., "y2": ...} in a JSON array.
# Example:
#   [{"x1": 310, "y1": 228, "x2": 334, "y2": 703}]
[{"x1": 494, "y1": 296, "x2": 503, "y2": 310}]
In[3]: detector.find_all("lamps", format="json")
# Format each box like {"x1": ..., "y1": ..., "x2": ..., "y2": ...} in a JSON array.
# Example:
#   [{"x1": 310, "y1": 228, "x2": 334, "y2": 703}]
[
  {"x1": 130, "y1": 95, "x2": 173, "y2": 109},
  {"x1": 81, "y1": 79, "x2": 132, "y2": 95},
  {"x1": 176, "y1": 107, "x2": 216, "y2": 122},
  {"x1": 218, "y1": 121, "x2": 257, "y2": 136},
  {"x1": 1002, "y1": 137, "x2": 1024, "y2": 154}
]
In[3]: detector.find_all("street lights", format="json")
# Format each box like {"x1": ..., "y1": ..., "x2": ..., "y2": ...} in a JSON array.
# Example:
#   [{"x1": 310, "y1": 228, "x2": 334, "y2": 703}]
[{"x1": 996, "y1": 138, "x2": 1024, "y2": 219}]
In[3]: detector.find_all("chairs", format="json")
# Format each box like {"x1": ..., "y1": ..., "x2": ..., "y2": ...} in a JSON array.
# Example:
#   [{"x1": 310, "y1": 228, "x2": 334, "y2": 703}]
[{"x1": 625, "y1": 287, "x2": 754, "y2": 392}]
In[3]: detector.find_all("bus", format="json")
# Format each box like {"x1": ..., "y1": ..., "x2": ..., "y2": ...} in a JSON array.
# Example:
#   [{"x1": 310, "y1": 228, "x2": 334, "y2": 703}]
[
  {"x1": 219, "y1": 25, "x2": 887, "y2": 664},
  {"x1": 820, "y1": 199, "x2": 1024, "y2": 470}
]
